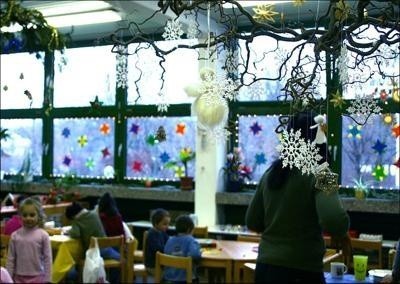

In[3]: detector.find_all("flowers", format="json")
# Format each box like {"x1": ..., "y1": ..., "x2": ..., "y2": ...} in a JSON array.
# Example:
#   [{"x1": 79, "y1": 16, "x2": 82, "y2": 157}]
[
  {"x1": 222, "y1": 154, "x2": 253, "y2": 181},
  {"x1": 177, "y1": 148, "x2": 195, "y2": 177}
]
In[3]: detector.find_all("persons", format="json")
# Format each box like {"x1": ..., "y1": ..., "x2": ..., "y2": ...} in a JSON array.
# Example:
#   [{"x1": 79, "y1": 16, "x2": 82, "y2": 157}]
[
  {"x1": 245, "y1": 110, "x2": 354, "y2": 284},
  {"x1": 145, "y1": 208, "x2": 171, "y2": 276},
  {"x1": 99, "y1": 191, "x2": 128, "y2": 252},
  {"x1": 163, "y1": 214, "x2": 202, "y2": 284},
  {"x1": 64, "y1": 203, "x2": 122, "y2": 277},
  {"x1": 380, "y1": 237, "x2": 400, "y2": 283},
  {"x1": 5, "y1": 196, "x2": 52, "y2": 283},
  {"x1": 4, "y1": 196, "x2": 27, "y2": 235}
]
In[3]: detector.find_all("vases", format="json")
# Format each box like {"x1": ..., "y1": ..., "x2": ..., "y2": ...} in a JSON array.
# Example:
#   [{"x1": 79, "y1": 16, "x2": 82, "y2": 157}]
[
  {"x1": 179, "y1": 177, "x2": 193, "y2": 190},
  {"x1": 226, "y1": 180, "x2": 245, "y2": 192}
]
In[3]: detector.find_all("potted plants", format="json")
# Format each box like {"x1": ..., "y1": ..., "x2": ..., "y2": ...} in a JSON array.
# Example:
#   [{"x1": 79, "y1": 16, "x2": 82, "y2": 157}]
[
  {"x1": 349, "y1": 178, "x2": 370, "y2": 199},
  {"x1": 4, "y1": 152, "x2": 34, "y2": 182}
]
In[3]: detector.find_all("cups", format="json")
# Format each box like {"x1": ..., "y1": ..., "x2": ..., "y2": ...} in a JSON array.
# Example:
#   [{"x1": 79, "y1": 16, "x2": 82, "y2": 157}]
[
  {"x1": 330, "y1": 262, "x2": 348, "y2": 279},
  {"x1": 353, "y1": 255, "x2": 368, "y2": 282}
]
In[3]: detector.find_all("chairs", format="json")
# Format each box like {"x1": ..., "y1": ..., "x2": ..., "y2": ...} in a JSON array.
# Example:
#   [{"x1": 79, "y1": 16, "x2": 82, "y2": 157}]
[
  {"x1": 89, "y1": 235, "x2": 125, "y2": 283},
  {"x1": 154, "y1": 251, "x2": 193, "y2": 284},
  {"x1": 347, "y1": 238, "x2": 383, "y2": 271}
]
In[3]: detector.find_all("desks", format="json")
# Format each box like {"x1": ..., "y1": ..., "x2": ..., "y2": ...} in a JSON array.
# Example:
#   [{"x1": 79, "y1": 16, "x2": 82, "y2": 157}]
[
  {"x1": 1, "y1": 202, "x2": 399, "y2": 259},
  {"x1": 196, "y1": 239, "x2": 336, "y2": 284}
]
